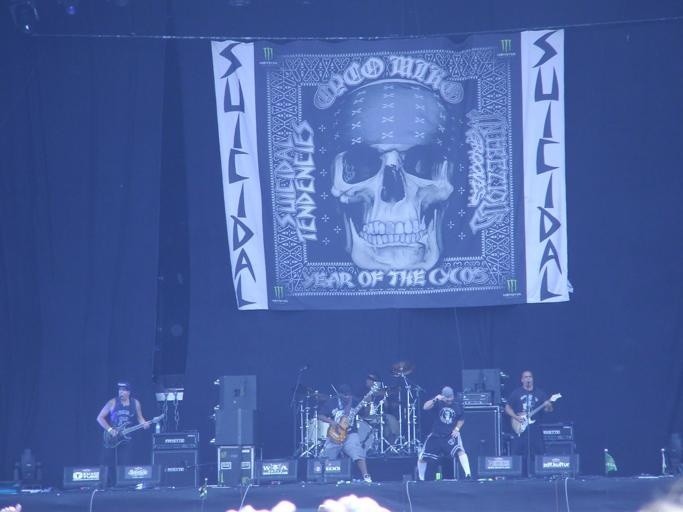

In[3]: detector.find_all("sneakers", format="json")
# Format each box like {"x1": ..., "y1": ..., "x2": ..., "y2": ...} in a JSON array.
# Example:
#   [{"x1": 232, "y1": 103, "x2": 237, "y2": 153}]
[
  {"x1": 465, "y1": 474, "x2": 472, "y2": 481},
  {"x1": 363, "y1": 474, "x2": 372, "y2": 483}
]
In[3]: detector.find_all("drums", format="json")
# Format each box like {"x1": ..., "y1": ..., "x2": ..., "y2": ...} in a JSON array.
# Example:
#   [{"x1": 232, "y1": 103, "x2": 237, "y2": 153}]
[{"x1": 366, "y1": 411, "x2": 404, "y2": 452}]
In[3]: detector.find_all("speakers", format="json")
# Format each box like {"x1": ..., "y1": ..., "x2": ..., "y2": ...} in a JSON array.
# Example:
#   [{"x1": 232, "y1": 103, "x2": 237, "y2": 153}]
[
  {"x1": 257, "y1": 458, "x2": 298, "y2": 484},
  {"x1": 217, "y1": 446, "x2": 262, "y2": 487},
  {"x1": 307, "y1": 458, "x2": 351, "y2": 484},
  {"x1": 534, "y1": 453, "x2": 580, "y2": 477},
  {"x1": 112, "y1": 464, "x2": 161, "y2": 491},
  {"x1": 63, "y1": 464, "x2": 108, "y2": 493},
  {"x1": 477, "y1": 455, "x2": 522, "y2": 478},
  {"x1": 454, "y1": 406, "x2": 507, "y2": 482},
  {"x1": 152, "y1": 449, "x2": 198, "y2": 488},
  {"x1": 219, "y1": 375, "x2": 256, "y2": 411},
  {"x1": 215, "y1": 408, "x2": 254, "y2": 445}
]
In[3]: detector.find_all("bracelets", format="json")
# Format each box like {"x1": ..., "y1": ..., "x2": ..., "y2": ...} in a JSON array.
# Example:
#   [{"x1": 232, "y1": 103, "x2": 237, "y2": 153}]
[
  {"x1": 453, "y1": 426, "x2": 460, "y2": 431},
  {"x1": 107, "y1": 427, "x2": 114, "y2": 432}
]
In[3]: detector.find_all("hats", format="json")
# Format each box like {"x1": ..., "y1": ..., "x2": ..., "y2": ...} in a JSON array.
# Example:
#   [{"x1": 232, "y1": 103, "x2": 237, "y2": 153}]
[{"x1": 442, "y1": 387, "x2": 454, "y2": 397}]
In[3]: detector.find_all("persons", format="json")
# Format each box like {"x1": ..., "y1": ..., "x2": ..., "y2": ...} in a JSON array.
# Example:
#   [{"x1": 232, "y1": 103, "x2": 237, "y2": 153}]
[
  {"x1": 97, "y1": 379, "x2": 150, "y2": 485},
  {"x1": 0, "y1": 503, "x2": 22, "y2": 512},
  {"x1": 316, "y1": 495, "x2": 387, "y2": 512},
  {"x1": 415, "y1": 385, "x2": 472, "y2": 480},
  {"x1": 226, "y1": 499, "x2": 299, "y2": 511},
  {"x1": 353, "y1": 369, "x2": 401, "y2": 444},
  {"x1": 505, "y1": 370, "x2": 555, "y2": 473},
  {"x1": 393, "y1": 352, "x2": 421, "y2": 420},
  {"x1": 317, "y1": 384, "x2": 372, "y2": 483}
]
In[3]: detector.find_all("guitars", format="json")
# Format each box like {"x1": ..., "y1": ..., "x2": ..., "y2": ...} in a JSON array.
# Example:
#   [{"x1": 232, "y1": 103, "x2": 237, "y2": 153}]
[
  {"x1": 102, "y1": 414, "x2": 164, "y2": 448},
  {"x1": 327, "y1": 383, "x2": 381, "y2": 444},
  {"x1": 511, "y1": 393, "x2": 562, "y2": 434}
]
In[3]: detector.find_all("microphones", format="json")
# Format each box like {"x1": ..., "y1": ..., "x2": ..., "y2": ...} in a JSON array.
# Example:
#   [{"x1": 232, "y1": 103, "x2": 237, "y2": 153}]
[{"x1": 295, "y1": 364, "x2": 310, "y2": 373}]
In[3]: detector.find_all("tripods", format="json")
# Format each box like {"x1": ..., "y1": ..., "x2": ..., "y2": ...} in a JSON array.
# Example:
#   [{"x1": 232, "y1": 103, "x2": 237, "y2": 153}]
[
  {"x1": 298, "y1": 404, "x2": 322, "y2": 458},
  {"x1": 393, "y1": 385, "x2": 422, "y2": 453},
  {"x1": 366, "y1": 388, "x2": 398, "y2": 456}
]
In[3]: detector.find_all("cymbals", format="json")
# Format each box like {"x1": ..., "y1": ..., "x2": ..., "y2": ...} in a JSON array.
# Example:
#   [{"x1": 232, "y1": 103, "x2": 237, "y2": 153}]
[
  {"x1": 389, "y1": 360, "x2": 415, "y2": 378},
  {"x1": 304, "y1": 393, "x2": 328, "y2": 402},
  {"x1": 374, "y1": 386, "x2": 399, "y2": 397}
]
